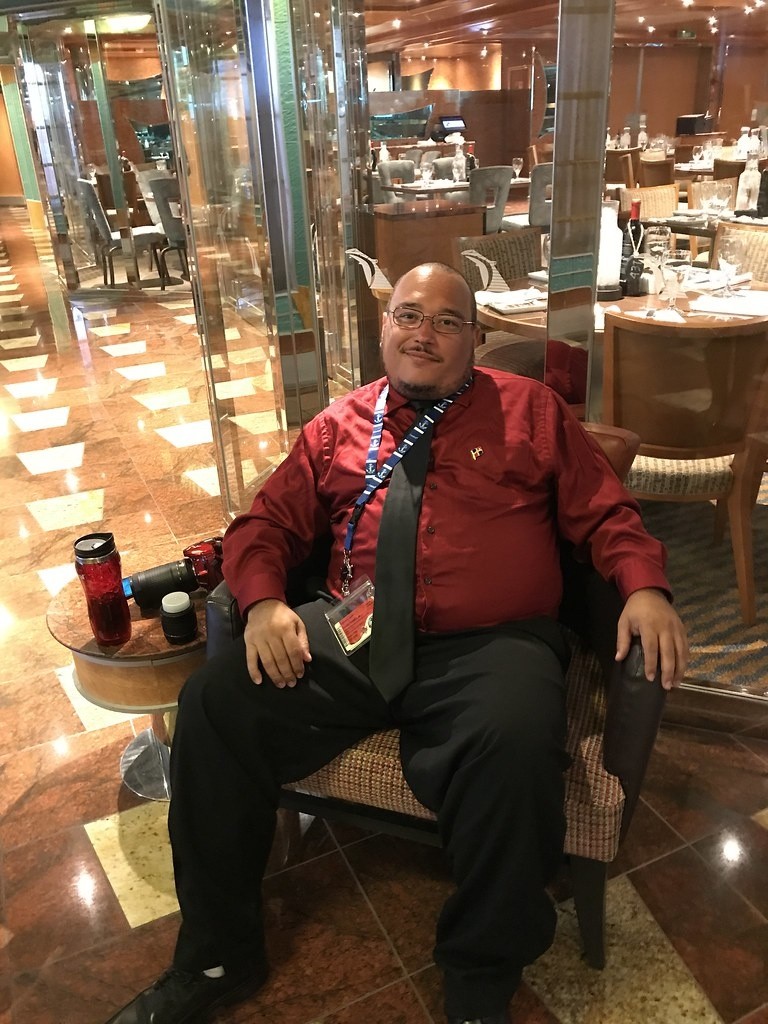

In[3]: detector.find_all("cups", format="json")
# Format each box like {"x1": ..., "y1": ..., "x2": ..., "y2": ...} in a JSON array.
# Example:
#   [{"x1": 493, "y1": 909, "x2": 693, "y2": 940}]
[
  {"x1": 543, "y1": 233, "x2": 550, "y2": 276},
  {"x1": 73, "y1": 532, "x2": 131, "y2": 645}
]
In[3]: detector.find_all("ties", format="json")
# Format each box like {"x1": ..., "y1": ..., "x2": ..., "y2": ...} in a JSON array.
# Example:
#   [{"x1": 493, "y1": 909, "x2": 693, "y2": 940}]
[{"x1": 368, "y1": 400, "x2": 441, "y2": 705}]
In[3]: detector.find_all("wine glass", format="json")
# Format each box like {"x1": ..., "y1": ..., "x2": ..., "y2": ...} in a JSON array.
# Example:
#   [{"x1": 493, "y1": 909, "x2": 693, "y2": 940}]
[
  {"x1": 370, "y1": 139, "x2": 479, "y2": 189},
  {"x1": 512, "y1": 157, "x2": 524, "y2": 182},
  {"x1": 715, "y1": 235, "x2": 743, "y2": 296},
  {"x1": 647, "y1": 225, "x2": 671, "y2": 285},
  {"x1": 660, "y1": 248, "x2": 692, "y2": 316},
  {"x1": 87, "y1": 163, "x2": 97, "y2": 183},
  {"x1": 606, "y1": 125, "x2": 766, "y2": 222}
]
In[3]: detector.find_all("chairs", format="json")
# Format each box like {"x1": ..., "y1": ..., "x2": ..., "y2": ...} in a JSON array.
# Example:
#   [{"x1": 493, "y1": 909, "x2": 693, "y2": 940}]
[
  {"x1": 202, "y1": 127, "x2": 768, "y2": 976},
  {"x1": 149, "y1": 177, "x2": 190, "y2": 292},
  {"x1": 94, "y1": 170, "x2": 175, "y2": 291},
  {"x1": 77, "y1": 178, "x2": 172, "y2": 291}
]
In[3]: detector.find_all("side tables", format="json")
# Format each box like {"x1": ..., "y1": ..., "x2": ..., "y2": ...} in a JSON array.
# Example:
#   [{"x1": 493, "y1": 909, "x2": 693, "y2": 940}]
[{"x1": 45, "y1": 564, "x2": 210, "y2": 805}]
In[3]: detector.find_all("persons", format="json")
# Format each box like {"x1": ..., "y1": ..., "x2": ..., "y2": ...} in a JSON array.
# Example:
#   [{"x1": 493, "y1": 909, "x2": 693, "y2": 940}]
[{"x1": 106, "y1": 261, "x2": 692, "y2": 1024}]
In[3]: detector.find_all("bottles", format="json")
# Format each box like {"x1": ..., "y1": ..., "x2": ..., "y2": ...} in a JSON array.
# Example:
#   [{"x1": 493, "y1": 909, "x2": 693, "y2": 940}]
[
  {"x1": 619, "y1": 198, "x2": 647, "y2": 297},
  {"x1": 160, "y1": 591, "x2": 198, "y2": 644},
  {"x1": 597, "y1": 200, "x2": 622, "y2": 289}
]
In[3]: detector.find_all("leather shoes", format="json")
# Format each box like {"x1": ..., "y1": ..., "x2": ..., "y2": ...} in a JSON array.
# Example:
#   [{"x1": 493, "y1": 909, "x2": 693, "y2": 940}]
[
  {"x1": 447, "y1": 1009, "x2": 512, "y2": 1024},
  {"x1": 105, "y1": 964, "x2": 267, "y2": 1024}
]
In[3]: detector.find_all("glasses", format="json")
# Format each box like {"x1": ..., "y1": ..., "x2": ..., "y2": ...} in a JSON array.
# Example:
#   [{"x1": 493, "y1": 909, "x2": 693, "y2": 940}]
[{"x1": 386, "y1": 306, "x2": 475, "y2": 335}]
